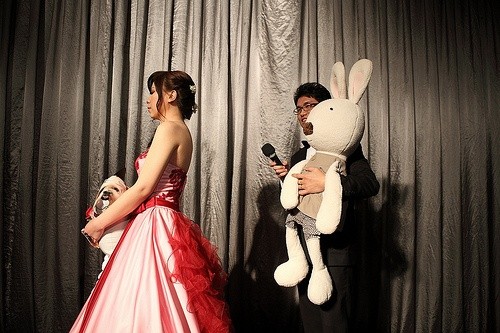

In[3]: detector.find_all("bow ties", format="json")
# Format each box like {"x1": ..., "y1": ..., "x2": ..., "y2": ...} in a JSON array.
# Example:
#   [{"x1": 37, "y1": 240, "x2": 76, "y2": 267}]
[{"x1": 301, "y1": 140, "x2": 310, "y2": 147}]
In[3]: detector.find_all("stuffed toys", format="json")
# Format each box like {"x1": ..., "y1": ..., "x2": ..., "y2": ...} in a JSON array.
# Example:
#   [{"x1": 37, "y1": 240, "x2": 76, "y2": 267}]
[
  {"x1": 274, "y1": 59, "x2": 373, "y2": 306},
  {"x1": 86, "y1": 168, "x2": 131, "y2": 279}
]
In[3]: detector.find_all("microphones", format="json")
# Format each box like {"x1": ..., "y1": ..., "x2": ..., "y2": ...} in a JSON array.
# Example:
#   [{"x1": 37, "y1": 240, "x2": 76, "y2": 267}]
[{"x1": 262, "y1": 143, "x2": 283, "y2": 166}]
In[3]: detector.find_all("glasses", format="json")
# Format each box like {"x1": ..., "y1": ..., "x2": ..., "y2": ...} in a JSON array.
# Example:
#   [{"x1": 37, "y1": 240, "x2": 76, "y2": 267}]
[{"x1": 293, "y1": 102, "x2": 319, "y2": 114}]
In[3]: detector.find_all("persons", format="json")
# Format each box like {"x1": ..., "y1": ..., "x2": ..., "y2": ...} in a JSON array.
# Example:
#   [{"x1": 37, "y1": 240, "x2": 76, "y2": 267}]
[
  {"x1": 268, "y1": 83, "x2": 380, "y2": 333},
  {"x1": 79, "y1": 70, "x2": 229, "y2": 333}
]
